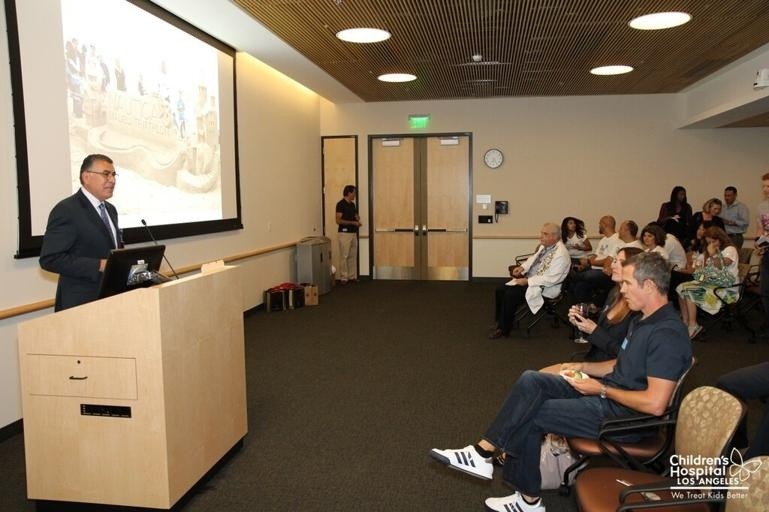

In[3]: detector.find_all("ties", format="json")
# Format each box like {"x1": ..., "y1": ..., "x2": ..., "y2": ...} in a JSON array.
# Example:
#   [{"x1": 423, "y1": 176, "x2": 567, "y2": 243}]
[{"x1": 99, "y1": 203, "x2": 115, "y2": 247}]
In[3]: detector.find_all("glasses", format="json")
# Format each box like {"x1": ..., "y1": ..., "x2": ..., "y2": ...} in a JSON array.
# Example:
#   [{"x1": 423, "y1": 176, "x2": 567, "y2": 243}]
[
  {"x1": 709, "y1": 199, "x2": 718, "y2": 208},
  {"x1": 87, "y1": 171, "x2": 119, "y2": 178},
  {"x1": 612, "y1": 257, "x2": 625, "y2": 264}
]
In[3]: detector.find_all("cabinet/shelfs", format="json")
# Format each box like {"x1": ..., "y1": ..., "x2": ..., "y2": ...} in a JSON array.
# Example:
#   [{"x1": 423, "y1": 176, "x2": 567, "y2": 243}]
[{"x1": 296, "y1": 235, "x2": 334, "y2": 296}]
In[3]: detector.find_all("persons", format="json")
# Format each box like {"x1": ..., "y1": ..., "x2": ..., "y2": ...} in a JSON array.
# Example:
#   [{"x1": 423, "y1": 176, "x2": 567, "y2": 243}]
[
  {"x1": 430, "y1": 173, "x2": 769, "y2": 512},
  {"x1": 67, "y1": 36, "x2": 219, "y2": 139},
  {"x1": 39, "y1": 153, "x2": 123, "y2": 315},
  {"x1": 336, "y1": 185, "x2": 363, "y2": 285}
]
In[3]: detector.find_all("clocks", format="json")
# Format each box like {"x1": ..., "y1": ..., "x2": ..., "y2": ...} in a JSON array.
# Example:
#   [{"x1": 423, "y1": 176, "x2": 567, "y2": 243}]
[{"x1": 483, "y1": 148, "x2": 504, "y2": 170}]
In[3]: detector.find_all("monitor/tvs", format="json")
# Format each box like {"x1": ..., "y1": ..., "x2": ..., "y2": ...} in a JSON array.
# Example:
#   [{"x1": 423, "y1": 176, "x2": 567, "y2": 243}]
[{"x1": 96, "y1": 244, "x2": 166, "y2": 300}]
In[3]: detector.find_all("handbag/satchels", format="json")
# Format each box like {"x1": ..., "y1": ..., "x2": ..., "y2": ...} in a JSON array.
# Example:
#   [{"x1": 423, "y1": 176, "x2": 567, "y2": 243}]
[
  {"x1": 692, "y1": 263, "x2": 735, "y2": 287},
  {"x1": 540, "y1": 433, "x2": 588, "y2": 490}
]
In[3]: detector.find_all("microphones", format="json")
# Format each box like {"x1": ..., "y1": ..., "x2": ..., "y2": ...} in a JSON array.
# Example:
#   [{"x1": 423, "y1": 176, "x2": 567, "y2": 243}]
[{"x1": 140, "y1": 219, "x2": 180, "y2": 279}]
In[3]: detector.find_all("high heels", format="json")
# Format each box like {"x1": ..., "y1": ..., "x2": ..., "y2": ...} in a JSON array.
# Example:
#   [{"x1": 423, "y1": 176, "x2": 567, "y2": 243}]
[{"x1": 688, "y1": 323, "x2": 703, "y2": 340}]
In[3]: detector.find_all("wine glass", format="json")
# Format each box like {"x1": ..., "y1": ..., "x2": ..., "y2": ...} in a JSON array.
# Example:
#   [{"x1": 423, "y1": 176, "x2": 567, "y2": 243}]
[{"x1": 573, "y1": 303, "x2": 589, "y2": 344}]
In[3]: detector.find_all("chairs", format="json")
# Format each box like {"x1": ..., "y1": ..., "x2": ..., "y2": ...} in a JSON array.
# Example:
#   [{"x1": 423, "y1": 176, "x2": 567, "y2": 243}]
[
  {"x1": 556, "y1": 352, "x2": 695, "y2": 499},
  {"x1": 573, "y1": 384, "x2": 747, "y2": 512},
  {"x1": 616, "y1": 454, "x2": 768, "y2": 512},
  {"x1": 505, "y1": 232, "x2": 769, "y2": 346}
]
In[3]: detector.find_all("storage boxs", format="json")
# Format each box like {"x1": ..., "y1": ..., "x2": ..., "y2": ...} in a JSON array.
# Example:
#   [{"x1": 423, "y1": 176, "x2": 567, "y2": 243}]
[{"x1": 304, "y1": 284, "x2": 318, "y2": 306}]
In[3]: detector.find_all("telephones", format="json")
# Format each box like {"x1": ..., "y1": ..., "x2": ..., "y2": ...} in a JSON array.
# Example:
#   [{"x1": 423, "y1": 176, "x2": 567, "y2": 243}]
[{"x1": 495, "y1": 201, "x2": 508, "y2": 223}]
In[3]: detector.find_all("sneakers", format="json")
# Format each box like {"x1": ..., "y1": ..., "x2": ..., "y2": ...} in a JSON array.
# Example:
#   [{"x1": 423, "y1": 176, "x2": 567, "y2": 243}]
[
  {"x1": 430, "y1": 444, "x2": 494, "y2": 481},
  {"x1": 489, "y1": 328, "x2": 509, "y2": 339},
  {"x1": 484, "y1": 492, "x2": 545, "y2": 512}
]
